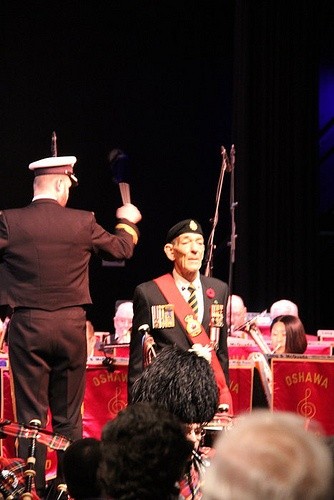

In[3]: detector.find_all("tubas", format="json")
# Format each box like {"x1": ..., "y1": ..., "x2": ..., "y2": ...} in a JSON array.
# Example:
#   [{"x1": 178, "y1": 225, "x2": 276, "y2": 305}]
[{"x1": 233, "y1": 310, "x2": 281, "y2": 411}]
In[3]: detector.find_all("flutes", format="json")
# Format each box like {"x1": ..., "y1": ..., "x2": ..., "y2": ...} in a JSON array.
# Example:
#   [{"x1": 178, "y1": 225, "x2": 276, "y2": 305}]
[
  {"x1": 0, "y1": 416, "x2": 73, "y2": 499},
  {"x1": 192, "y1": 449, "x2": 209, "y2": 479}
]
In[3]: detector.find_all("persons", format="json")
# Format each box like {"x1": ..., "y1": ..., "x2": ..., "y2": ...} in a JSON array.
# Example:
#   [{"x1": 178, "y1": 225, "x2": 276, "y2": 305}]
[
  {"x1": 103, "y1": 402, "x2": 200, "y2": 500},
  {"x1": 226, "y1": 295, "x2": 247, "y2": 330},
  {"x1": 114, "y1": 301, "x2": 134, "y2": 345},
  {"x1": 200, "y1": 410, "x2": 334, "y2": 500},
  {"x1": 270, "y1": 299, "x2": 298, "y2": 323},
  {"x1": 62, "y1": 437, "x2": 108, "y2": 500},
  {"x1": 128, "y1": 218, "x2": 234, "y2": 419},
  {"x1": 0, "y1": 155, "x2": 143, "y2": 500},
  {"x1": 269, "y1": 314, "x2": 307, "y2": 353},
  {"x1": 85, "y1": 321, "x2": 115, "y2": 357},
  {"x1": 133, "y1": 343, "x2": 220, "y2": 500}
]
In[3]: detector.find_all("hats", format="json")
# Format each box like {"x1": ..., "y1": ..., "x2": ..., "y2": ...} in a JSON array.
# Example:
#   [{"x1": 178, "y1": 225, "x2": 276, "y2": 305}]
[
  {"x1": 29, "y1": 156, "x2": 78, "y2": 186},
  {"x1": 129, "y1": 347, "x2": 220, "y2": 424},
  {"x1": 166, "y1": 219, "x2": 203, "y2": 244}
]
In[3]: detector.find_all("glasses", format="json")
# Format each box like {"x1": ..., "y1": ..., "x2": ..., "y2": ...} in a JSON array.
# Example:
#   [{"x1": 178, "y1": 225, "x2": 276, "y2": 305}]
[{"x1": 182, "y1": 428, "x2": 206, "y2": 441}]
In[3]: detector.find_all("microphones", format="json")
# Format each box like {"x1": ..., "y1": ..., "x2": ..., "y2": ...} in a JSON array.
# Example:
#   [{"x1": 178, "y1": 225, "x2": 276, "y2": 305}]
[
  {"x1": 221, "y1": 146, "x2": 232, "y2": 172},
  {"x1": 51, "y1": 132, "x2": 57, "y2": 157}
]
